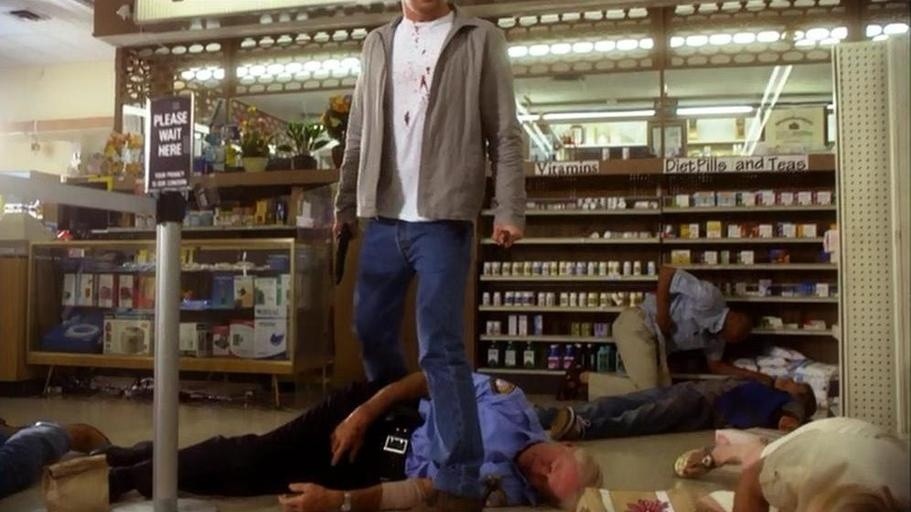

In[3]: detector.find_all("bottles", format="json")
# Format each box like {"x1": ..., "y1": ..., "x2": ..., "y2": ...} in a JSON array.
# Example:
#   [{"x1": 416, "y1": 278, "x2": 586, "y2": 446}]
[{"x1": 482, "y1": 260, "x2": 657, "y2": 373}]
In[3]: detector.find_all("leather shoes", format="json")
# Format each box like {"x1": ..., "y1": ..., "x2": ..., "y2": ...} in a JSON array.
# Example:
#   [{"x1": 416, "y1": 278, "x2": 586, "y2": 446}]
[
  {"x1": 62, "y1": 423, "x2": 111, "y2": 451},
  {"x1": 91, "y1": 441, "x2": 148, "y2": 463},
  {"x1": 107, "y1": 469, "x2": 124, "y2": 498}
]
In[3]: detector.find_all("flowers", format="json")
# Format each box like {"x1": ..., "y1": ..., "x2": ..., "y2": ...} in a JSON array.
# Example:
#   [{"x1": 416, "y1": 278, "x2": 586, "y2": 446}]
[
  {"x1": 321, "y1": 95, "x2": 352, "y2": 145},
  {"x1": 232, "y1": 107, "x2": 281, "y2": 156}
]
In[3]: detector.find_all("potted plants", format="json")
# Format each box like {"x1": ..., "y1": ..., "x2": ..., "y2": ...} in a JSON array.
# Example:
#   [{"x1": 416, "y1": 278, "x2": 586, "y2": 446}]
[{"x1": 277, "y1": 121, "x2": 330, "y2": 169}]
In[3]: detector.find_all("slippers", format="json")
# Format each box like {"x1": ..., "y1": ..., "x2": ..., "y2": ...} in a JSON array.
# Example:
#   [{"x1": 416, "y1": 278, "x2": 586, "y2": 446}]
[{"x1": 673, "y1": 446, "x2": 718, "y2": 479}]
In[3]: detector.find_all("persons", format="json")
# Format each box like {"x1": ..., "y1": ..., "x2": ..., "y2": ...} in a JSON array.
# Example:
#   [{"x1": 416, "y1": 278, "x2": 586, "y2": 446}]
[
  {"x1": 0, "y1": 416, "x2": 113, "y2": 499},
  {"x1": 551, "y1": 373, "x2": 818, "y2": 441},
  {"x1": 557, "y1": 264, "x2": 768, "y2": 404},
  {"x1": 89, "y1": 370, "x2": 605, "y2": 512},
  {"x1": 329, "y1": 0, "x2": 527, "y2": 512},
  {"x1": 674, "y1": 415, "x2": 911, "y2": 512}
]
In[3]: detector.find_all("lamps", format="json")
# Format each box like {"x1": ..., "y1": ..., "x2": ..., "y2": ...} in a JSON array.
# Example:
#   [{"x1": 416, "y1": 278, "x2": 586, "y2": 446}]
[
  {"x1": 543, "y1": 105, "x2": 757, "y2": 123},
  {"x1": 517, "y1": 113, "x2": 541, "y2": 124}
]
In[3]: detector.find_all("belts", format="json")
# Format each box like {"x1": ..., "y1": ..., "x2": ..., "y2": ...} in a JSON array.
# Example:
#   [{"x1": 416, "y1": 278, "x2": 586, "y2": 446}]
[{"x1": 380, "y1": 418, "x2": 421, "y2": 478}]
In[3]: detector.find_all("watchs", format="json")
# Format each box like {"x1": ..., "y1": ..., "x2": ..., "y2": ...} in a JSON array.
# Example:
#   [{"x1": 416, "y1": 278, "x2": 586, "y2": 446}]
[{"x1": 340, "y1": 489, "x2": 352, "y2": 511}]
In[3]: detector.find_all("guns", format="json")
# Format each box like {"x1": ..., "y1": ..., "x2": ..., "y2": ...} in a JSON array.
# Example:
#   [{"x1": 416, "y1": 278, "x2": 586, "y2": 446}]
[{"x1": 333, "y1": 223, "x2": 353, "y2": 285}]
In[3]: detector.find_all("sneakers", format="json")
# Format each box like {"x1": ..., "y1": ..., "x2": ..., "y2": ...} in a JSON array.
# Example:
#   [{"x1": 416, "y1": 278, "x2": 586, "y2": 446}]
[
  {"x1": 551, "y1": 406, "x2": 589, "y2": 440},
  {"x1": 549, "y1": 362, "x2": 585, "y2": 402},
  {"x1": 407, "y1": 490, "x2": 482, "y2": 512}
]
